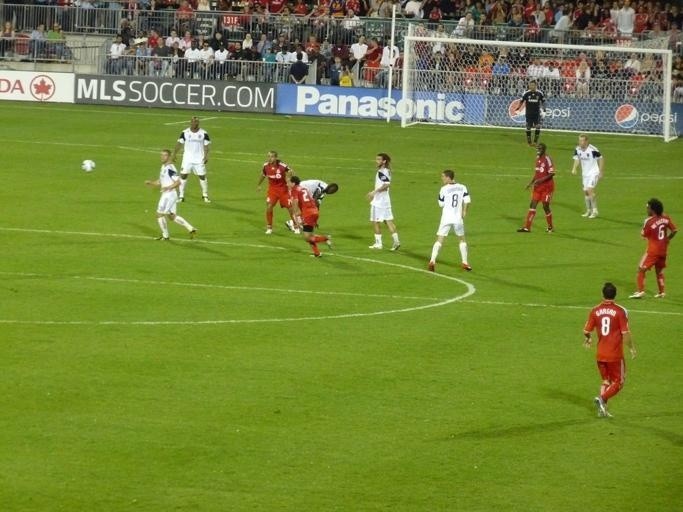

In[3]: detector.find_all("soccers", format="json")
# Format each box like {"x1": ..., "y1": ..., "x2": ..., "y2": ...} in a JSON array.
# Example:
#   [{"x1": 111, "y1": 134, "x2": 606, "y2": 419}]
[{"x1": 80, "y1": 159, "x2": 96, "y2": 172}]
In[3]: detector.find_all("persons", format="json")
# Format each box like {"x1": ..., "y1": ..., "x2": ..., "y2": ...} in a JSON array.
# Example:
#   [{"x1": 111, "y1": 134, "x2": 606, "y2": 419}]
[
  {"x1": 145, "y1": 0, "x2": 161, "y2": 28},
  {"x1": 211, "y1": 31, "x2": 228, "y2": 50},
  {"x1": 286, "y1": 176, "x2": 331, "y2": 258},
  {"x1": 291, "y1": 37, "x2": 305, "y2": 53},
  {"x1": 47, "y1": 22, "x2": 71, "y2": 63},
  {"x1": 627, "y1": 198, "x2": 678, "y2": 299},
  {"x1": 284, "y1": 180, "x2": 339, "y2": 234},
  {"x1": 30, "y1": 24, "x2": 49, "y2": 58},
  {"x1": 183, "y1": 39, "x2": 200, "y2": 78},
  {"x1": 196, "y1": 0, "x2": 268, "y2": 33},
  {"x1": 176, "y1": 1, "x2": 193, "y2": 29},
  {"x1": 514, "y1": 80, "x2": 547, "y2": 148},
  {"x1": 242, "y1": 33, "x2": 254, "y2": 51},
  {"x1": 426, "y1": 0, "x2": 683, "y2": 45},
  {"x1": 305, "y1": 35, "x2": 320, "y2": 53},
  {"x1": 571, "y1": 134, "x2": 603, "y2": 219},
  {"x1": 310, "y1": 45, "x2": 326, "y2": 86},
  {"x1": 120, "y1": 18, "x2": 133, "y2": 47},
  {"x1": 547, "y1": 54, "x2": 683, "y2": 102},
  {"x1": 122, "y1": 0, "x2": 141, "y2": 20},
  {"x1": 144, "y1": 149, "x2": 198, "y2": 241},
  {"x1": 255, "y1": 151, "x2": 301, "y2": 234},
  {"x1": 515, "y1": 46, "x2": 548, "y2": 80},
  {"x1": 583, "y1": 282, "x2": 636, "y2": 417},
  {"x1": 134, "y1": 30, "x2": 149, "y2": 48},
  {"x1": 109, "y1": 35, "x2": 127, "y2": 75},
  {"x1": 289, "y1": 52, "x2": 308, "y2": 85},
  {"x1": 292, "y1": 46, "x2": 309, "y2": 64},
  {"x1": 149, "y1": 37, "x2": 170, "y2": 79},
  {"x1": 148, "y1": 28, "x2": 161, "y2": 47},
  {"x1": 172, "y1": 116, "x2": 212, "y2": 204},
  {"x1": 362, "y1": 38, "x2": 382, "y2": 67},
  {"x1": 349, "y1": 34, "x2": 368, "y2": 63},
  {"x1": 199, "y1": 40, "x2": 214, "y2": 78},
  {"x1": 417, "y1": 44, "x2": 514, "y2": 93},
  {"x1": 268, "y1": 0, "x2": 425, "y2": 32},
  {"x1": 135, "y1": 42, "x2": 149, "y2": 77},
  {"x1": 165, "y1": 29, "x2": 180, "y2": 47},
  {"x1": 330, "y1": 56, "x2": 343, "y2": 85},
  {"x1": 179, "y1": 30, "x2": 192, "y2": 49},
  {"x1": 428, "y1": 170, "x2": 472, "y2": 272},
  {"x1": 517, "y1": 143, "x2": 556, "y2": 233},
  {"x1": 256, "y1": 34, "x2": 271, "y2": 62},
  {"x1": 272, "y1": 33, "x2": 292, "y2": 54},
  {"x1": 169, "y1": 41, "x2": 183, "y2": 80},
  {"x1": 365, "y1": 152, "x2": 400, "y2": 251},
  {"x1": 228, "y1": 42, "x2": 243, "y2": 76},
  {"x1": 375, "y1": 38, "x2": 400, "y2": 89},
  {"x1": 214, "y1": 42, "x2": 229, "y2": 81},
  {"x1": 0, "y1": 20, "x2": 15, "y2": 58},
  {"x1": 275, "y1": 44, "x2": 291, "y2": 67}
]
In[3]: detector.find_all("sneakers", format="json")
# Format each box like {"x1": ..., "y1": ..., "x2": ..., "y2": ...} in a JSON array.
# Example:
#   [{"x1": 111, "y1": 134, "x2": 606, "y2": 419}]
[
  {"x1": 461, "y1": 262, "x2": 472, "y2": 271},
  {"x1": 202, "y1": 197, "x2": 213, "y2": 203},
  {"x1": 599, "y1": 406, "x2": 614, "y2": 418},
  {"x1": 285, "y1": 220, "x2": 294, "y2": 231},
  {"x1": 368, "y1": 243, "x2": 382, "y2": 249},
  {"x1": 515, "y1": 227, "x2": 530, "y2": 232},
  {"x1": 589, "y1": 208, "x2": 598, "y2": 218},
  {"x1": 326, "y1": 235, "x2": 332, "y2": 250},
  {"x1": 265, "y1": 227, "x2": 273, "y2": 234},
  {"x1": 176, "y1": 197, "x2": 183, "y2": 202},
  {"x1": 545, "y1": 227, "x2": 552, "y2": 232},
  {"x1": 534, "y1": 142, "x2": 538, "y2": 148},
  {"x1": 390, "y1": 241, "x2": 400, "y2": 251},
  {"x1": 527, "y1": 142, "x2": 533, "y2": 148},
  {"x1": 294, "y1": 228, "x2": 301, "y2": 234},
  {"x1": 428, "y1": 262, "x2": 434, "y2": 272},
  {"x1": 594, "y1": 397, "x2": 606, "y2": 417},
  {"x1": 188, "y1": 228, "x2": 198, "y2": 239},
  {"x1": 153, "y1": 237, "x2": 169, "y2": 242},
  {"x1": 654, "y1": 293, "x2": 666, "y2": 298},
  {"x1": 310, "y1": 253, "x2": 322, "y2": 258},
  {"x1": 582, "y1": 209, "x2": 591, "y2": 217},
  {"x1": 629, "y1": 291, "x2": 645, "y2": 299}
]
